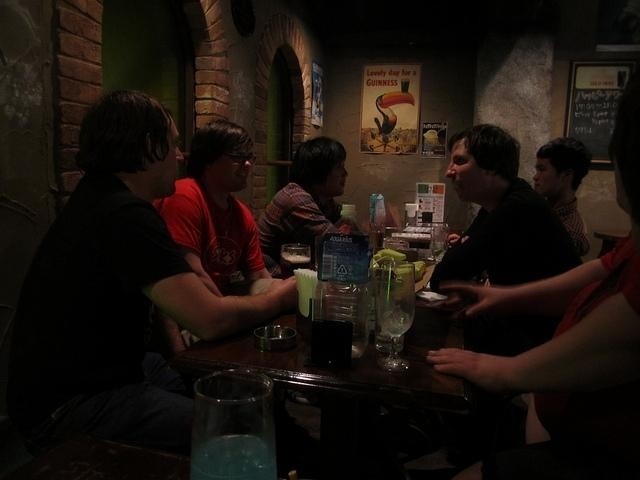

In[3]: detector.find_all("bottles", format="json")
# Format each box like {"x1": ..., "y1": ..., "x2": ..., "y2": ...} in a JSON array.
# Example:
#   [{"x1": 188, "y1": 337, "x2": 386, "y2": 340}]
[
  {"x1": 369, "y1": 194, "x2": 386, "y2": 227},
  {"x1": 318, "y1": 203, "x2": 370, "y2": 369},
  {"x1": 424, "y1": 130, "x2": 438, "y2": 151}
]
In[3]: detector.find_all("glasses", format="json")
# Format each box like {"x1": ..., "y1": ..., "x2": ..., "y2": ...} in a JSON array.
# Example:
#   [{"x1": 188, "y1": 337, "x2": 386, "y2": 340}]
[{"x1": 223, "y1": 150, "x2": 258, "y2": 166}]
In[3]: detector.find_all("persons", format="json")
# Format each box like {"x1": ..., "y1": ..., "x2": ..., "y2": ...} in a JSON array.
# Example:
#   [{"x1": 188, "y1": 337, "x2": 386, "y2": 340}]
[
  {"x1": 257, "y1": 137, "x2": 408, "y2": 268},
  {"x1": 154, "y1": 119, "x2": 287, "y2": 359},
  {"x1": 432, "y1": 122, "x2": 584, "y2": 357},
  {"x1": 6, "y1": 90, "x2": 300, "y2": 460},
  {"x1": 424, "y1": 70, "x2": 639, "y2": 478},
  {"x1": 444, "y1": 137, "x2": 592, "y2": 257}
]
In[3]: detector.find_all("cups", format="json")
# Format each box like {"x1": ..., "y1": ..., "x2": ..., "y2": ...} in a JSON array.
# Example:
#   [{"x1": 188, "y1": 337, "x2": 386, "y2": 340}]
[
  {"x1": 281, "y1": 245, "x2": 312, "y2": 284},
  {"x1": 189, "y1": 370, "x2": 277, "y2": 480},
  {"x1": 401, "y1": 79, "x2": 411, "y2": 92}
]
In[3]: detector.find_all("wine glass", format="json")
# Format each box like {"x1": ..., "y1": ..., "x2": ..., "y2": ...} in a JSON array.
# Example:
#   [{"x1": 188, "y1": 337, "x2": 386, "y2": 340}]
[
  {"x1": 367, "y1": 261, "x2": 416, "y2": 371},
  {"x1": 383, "y1": 223, "x2": 449, "y2": 270}
]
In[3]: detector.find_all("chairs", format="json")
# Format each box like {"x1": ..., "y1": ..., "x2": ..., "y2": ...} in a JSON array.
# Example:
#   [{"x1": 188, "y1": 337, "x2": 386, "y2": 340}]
[{"x1": 2, "y1": 261, "x2": 468, "y2": 478}]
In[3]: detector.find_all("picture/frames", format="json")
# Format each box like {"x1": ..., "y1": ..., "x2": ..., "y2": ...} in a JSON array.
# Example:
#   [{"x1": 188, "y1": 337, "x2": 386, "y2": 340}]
[{"x1": 564, "y1": 59, "x2": 634, "y2": 172}]
